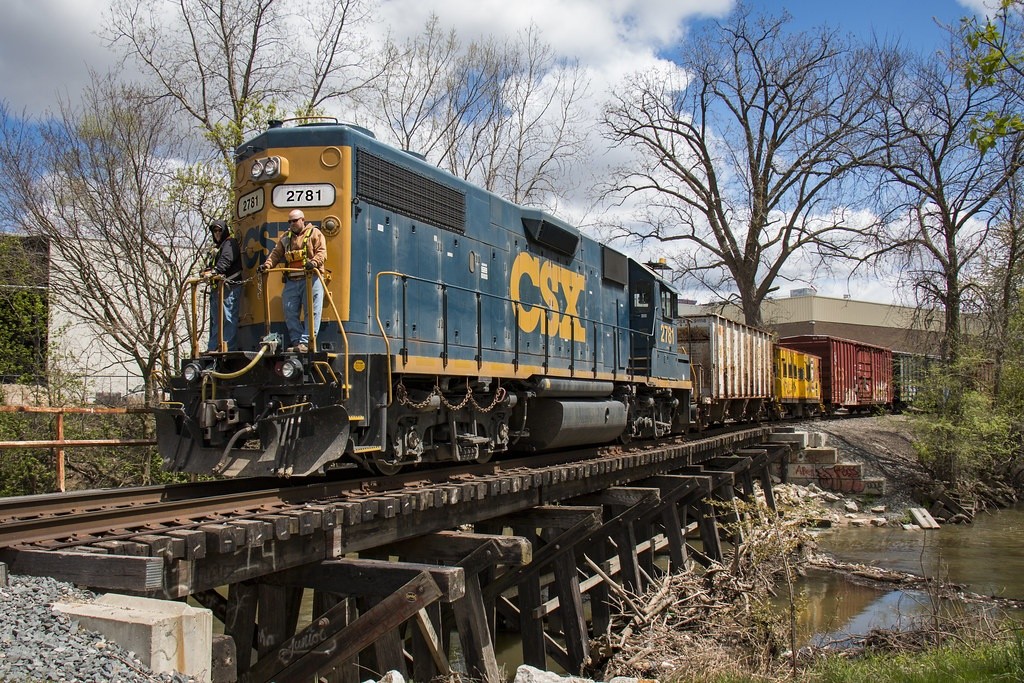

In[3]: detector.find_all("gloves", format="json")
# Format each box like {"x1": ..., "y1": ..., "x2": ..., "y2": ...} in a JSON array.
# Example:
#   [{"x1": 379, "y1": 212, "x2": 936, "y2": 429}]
[
  {"x1": 256, "y1": 265, "x2": 267, "y2": 273},
  {"x1": 302, "y1": 263, "x2": 312, "y2": 270}
]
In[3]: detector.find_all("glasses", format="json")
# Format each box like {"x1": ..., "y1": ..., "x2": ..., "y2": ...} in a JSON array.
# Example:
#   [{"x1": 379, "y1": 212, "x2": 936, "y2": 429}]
[
  {"x1": 214, "y1": 228, "x2": 222, "y2": 233},
  {"x1": 288, "y1": 217, "x2": 301, "y2": 223}
]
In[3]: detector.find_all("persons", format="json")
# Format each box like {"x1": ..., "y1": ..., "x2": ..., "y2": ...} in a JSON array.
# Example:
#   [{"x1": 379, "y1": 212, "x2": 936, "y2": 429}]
[
  {"x1": 258, "y1": 208, "x2": 330, "y2": 353},
  {"x1": 198, "y1": 219, "x2": 244, "y2": 354}
]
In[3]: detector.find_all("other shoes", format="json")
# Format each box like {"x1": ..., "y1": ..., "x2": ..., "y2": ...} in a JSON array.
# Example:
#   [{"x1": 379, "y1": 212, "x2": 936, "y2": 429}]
[
  {"x1": 287, "y1": 347, "x2": 292, "y2": 352},
  {"x1": 294, "y1": 344, "x2": 307, "y2": 352}
]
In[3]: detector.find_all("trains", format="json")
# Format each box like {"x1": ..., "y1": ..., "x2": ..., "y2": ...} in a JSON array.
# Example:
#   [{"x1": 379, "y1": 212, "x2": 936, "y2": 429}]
[{"x1": 150, "y1": 114, "x2": 998, "y2": 486}]
